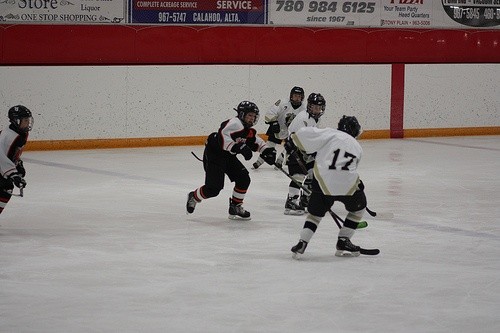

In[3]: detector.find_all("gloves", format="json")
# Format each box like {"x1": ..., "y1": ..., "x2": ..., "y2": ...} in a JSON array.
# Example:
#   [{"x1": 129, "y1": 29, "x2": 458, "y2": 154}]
[
  {"x1": 262, "y1": 148, "x2": 276, "y2": 165},
  {"x1": 13, "y1": 176, "x2": 26, "y2": 189},
  {"x1": 358, "y1": 180, "x2": 366, "y2": 191},
  {"x1": 235, "y1": 141, "x2": 253, "y2": 160},
  {"x1": 13, "y1": 160, "x2": 26, "y2": 178}
]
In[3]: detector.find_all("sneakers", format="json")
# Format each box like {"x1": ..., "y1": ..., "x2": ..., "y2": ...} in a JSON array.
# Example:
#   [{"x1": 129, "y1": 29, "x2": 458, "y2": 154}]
[
  {"x1": 291, "y1": 238, "x2": 308, "y2": 260},
  {"x1": 274, "y1": 163, "x2": 282, "y2": 171},
  {"x1": 284, "y1": 193, "x2": 305, "y2": 215},
  {"x1": 251, "y1": 162, "x2": 259, "y2": 169},
  {"x1": 228, "y1": 197, "x2": 251, "y2": 221},
  {"x1": 300, "y1": 191, "x2": 310, "y2": 213},
  {"x1": 186, "y1": 191, "x2": 196, "y2": 215},
  {"x1": 335, "y1": 236, "x2": 361, "y2": 257}
]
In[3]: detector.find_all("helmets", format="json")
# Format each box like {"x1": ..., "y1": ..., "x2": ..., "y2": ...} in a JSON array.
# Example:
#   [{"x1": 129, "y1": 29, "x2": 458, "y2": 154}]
[
  {"x1": 337, "y1": 115, "x2": 360, "y2": 138},
  {"x1": 8, "y1": 105, "x2": 34, "y2": 133},
  {"x1": 289, "y1": 87, "x2": 304, "y2": 102},
  {"x1": 306, "y1": 93, "x2": 326, "y2": 118},
  {"x1": 233, "y1": 101, "x2": 260, "y2": 127}
]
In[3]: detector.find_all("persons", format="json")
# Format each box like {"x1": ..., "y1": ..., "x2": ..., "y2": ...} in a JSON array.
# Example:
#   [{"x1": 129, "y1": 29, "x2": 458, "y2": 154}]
[
  {"x1": 284, "y1": 92, "x2": 327, "y2": 215},
  {"x1": 184, "y1": 100, "x2": 278, "y2": 220},
  {"x1": 253, "y1": 86, "x2": 305, "y2": 169},
  {"x1": 0, "y1": 105, "x2": 34, "y2": 213},
  {"x1": 289, "y1": 114, "x2": 368, "y2": 260}
]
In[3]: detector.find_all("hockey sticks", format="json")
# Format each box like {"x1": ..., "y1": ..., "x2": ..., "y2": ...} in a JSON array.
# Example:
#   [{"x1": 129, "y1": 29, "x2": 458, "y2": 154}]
[
  {"x1": 366, "y1": 208, "x2": 377, "y2": 217},
  {"x1": 274, "y1": 162, "x2": 368, "y2": 228},
  {"x1": 8, "y1": 182, "x2": 23, "y2": 197},
  {"x1": 291, "y1": 150, "x2": 381, "y2": 256}
]
